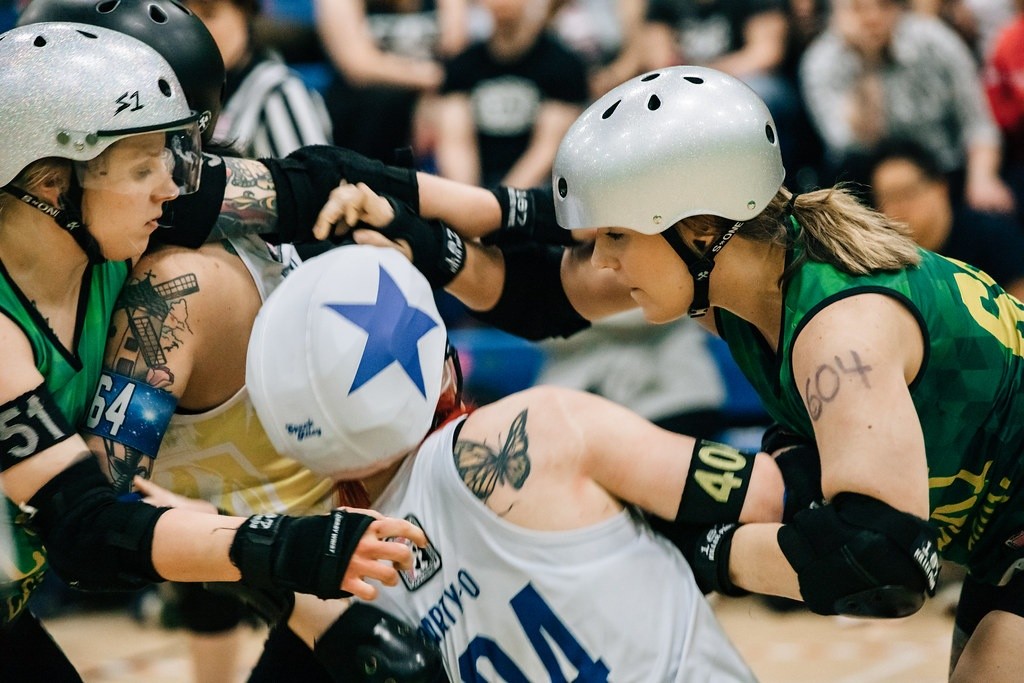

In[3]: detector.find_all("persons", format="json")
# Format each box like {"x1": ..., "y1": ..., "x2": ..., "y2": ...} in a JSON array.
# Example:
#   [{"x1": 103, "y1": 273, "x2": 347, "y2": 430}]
[{"x1": 0, "y1": 0, "x2": 1023, "y2": 683}]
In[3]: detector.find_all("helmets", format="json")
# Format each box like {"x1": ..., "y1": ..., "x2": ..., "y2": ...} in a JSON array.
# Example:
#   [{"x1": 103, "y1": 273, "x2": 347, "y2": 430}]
[
  {"x1": 245, "y1": 244, "x2": 448, "y2": 481},
  {"x1": 1, "y1": 23, "x2": 197, "y2": 189},
  {"x1": 34, "y1": 1, "x2": 226, "y2": 147},
  {"x1": 552, "y1": 64, "x2": 784, "y2": 235}
]
